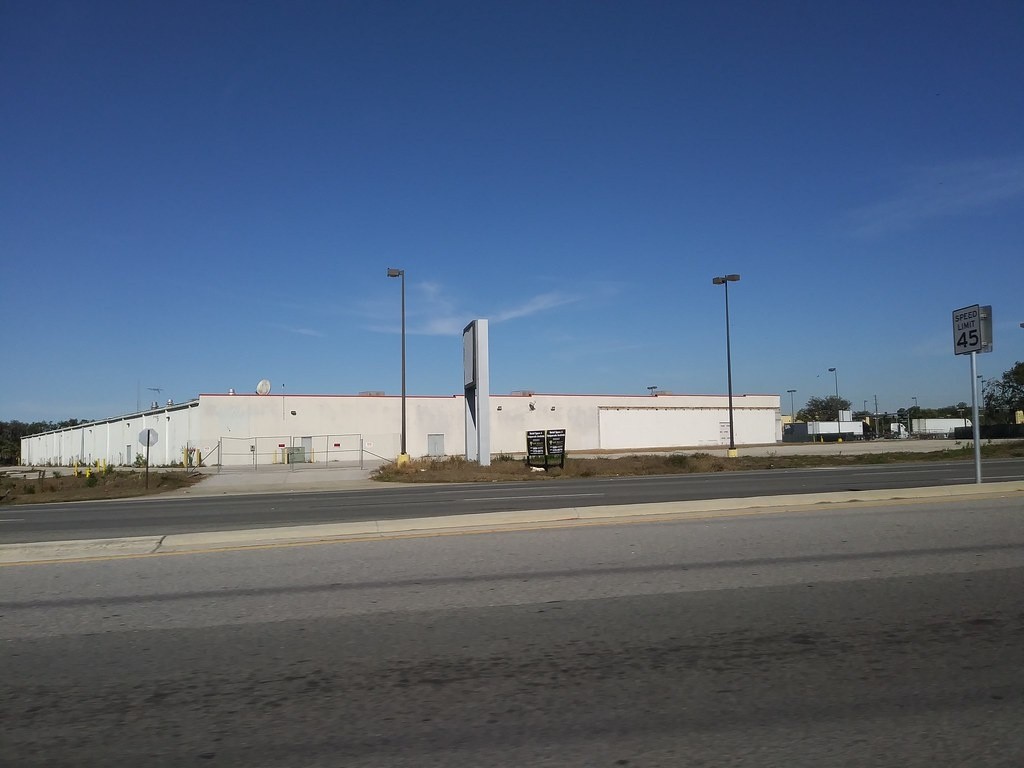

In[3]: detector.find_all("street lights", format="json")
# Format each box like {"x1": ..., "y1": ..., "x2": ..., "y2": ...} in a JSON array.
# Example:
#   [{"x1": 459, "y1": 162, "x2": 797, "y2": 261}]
[
  {"x1": 387, "y1": 268, "x2": 409, "y2": 469},
  {"x1": 786, "y1": 390, "x2": 796, "y2": 423},
  {"x1": 712, "y1": 274, "x2": 740, "y2": 457},
  {"x1": 829, "y1": 368, "x2": 838, "y2": 397},
  {"x1": 977, "y1": 374, "x2": 985, "y2": 413}
]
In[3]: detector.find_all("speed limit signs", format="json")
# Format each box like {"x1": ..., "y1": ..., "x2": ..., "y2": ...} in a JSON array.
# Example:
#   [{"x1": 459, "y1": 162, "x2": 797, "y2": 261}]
[{"x1": 952, "y1": 304, "x2": 993, "y2": 355}]
